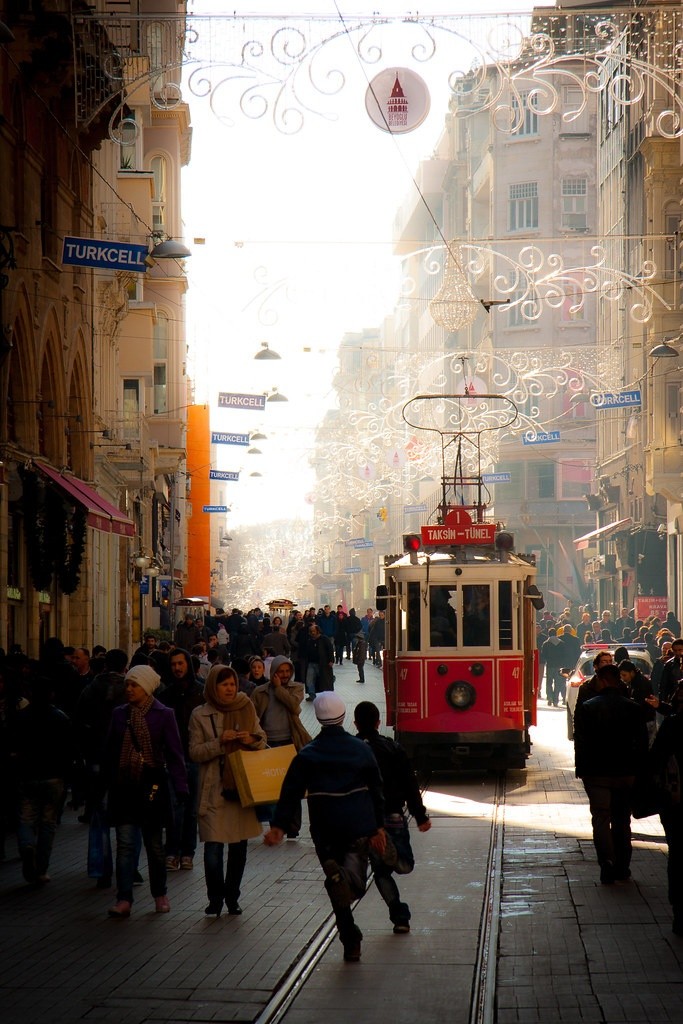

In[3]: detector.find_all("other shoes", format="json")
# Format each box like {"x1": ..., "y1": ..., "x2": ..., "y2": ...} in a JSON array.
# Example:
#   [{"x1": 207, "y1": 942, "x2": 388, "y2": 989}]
[
  {"x1": 156, "y1": 896, "x2": 169, "y2": 912},
  {"x1": 344, "y1": 925, "x2": 362, "y2": 961},
  {"x1": 286, "y1": 833, "x2": 299, "y2": 838},
  {"x1": 22, "y1": 846, "x2": 51, "y2": 882},
  {"x1": 378, "y1": 832, "x2": 397, "y2": 867},
  {"x1": 166, "y1": 855, "x2": 180, "y2": 872},
  {"x1": 181, "y1": 856, "x2": 194, "y2": 869},
  {"x1": 392, "y1": 919, "x2": 411, "y2": 932},
  {"x1": 132, "y1": 870, "x2": 144, "y2": 886},
  {"x1": 322, "y1": 859, "x2": 354, "y2": 907},
  {"x1": 108, "y1": 898, "x2": 131, "y2": 917},
  {"x1": 96, "y1": 877, "x2": 110, "y2": 888}
]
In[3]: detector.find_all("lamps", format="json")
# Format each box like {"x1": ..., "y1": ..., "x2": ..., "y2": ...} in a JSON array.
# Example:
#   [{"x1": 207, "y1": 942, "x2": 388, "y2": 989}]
[
  {"x1": 37, "y1": 411, "x2": 81, "y2": 422},
  {"x1": 7, "y1": 397, "x2": 56, "y2": 408},
  {"x1": 250, "y1": 471, "x2": 262, "y2": 476},
  {"x1": 358, "y1": 506, "x2": 371, "y2": 513},
  {"x1": 247, "y1": 448, "x2": 262, "y2": 454},
  {"x1": 128, "y1": 546, "x2": 160, "y2": 577},
  {"x1": 90, "y1": 441, "x2": 131, "y2": 450},
  {"x1": 569, "y1": 390, "x2": 591, "y2": 402},
  {"x1": 419, "y1": 473, "x2": 434, "y2": 481},
  {"x1": 268, "y1": 389, "x2": 289, "y2": 401},
  {"x1": 251, "y1": 431, "x2": 267, "y2": 439},
  {"x1": 223, "y1": 535, "x2": 232, "y2": 540},
  {"x1": 499, "y1": 430, "x2": 518, "y2": 441},
  {"x1": 65, "y1": 427, "x2": 108, "y2": 436},
  {"x1": 219, "y1": 541, "x2": 230, "y2": 546},
  {"x1": 650, "y1": 341, "x2": 680, "y2": 358},
  {"x1": 215, "y1": 558, "x2": 223, "y2": 562},
  {"x1": 151, "y1": 236, "x2": 192, "y2": 259},
  {"x1": 254, "y1": 345, "x2": 282, "y2": 360}
]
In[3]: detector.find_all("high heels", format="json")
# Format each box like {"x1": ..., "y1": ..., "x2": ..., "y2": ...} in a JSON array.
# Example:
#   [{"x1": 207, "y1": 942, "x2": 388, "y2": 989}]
[
  {"x1": 204, "y1": 897, "x2": 223, "y2": 916},
  {"x1": 225, "y1": 896, "x2": 243, "y2": 915}
]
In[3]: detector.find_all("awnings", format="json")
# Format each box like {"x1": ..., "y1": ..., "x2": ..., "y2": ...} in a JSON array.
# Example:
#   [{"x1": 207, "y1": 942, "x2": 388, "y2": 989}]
[
  {"x1": 33, "y1": 461, "x2": 135, "y2": 538},
  {"x1": 572, "y1": 517, "x2": 631, "y2": 551}
]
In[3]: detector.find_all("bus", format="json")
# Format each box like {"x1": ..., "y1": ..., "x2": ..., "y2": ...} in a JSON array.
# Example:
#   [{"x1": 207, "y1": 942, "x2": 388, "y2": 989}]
[{"x1": 372, "y1": 357, "x2": 543, "y2": 778}]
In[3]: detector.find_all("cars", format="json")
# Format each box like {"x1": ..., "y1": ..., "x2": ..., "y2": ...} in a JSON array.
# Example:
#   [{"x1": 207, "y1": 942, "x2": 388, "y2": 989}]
[{"x1": 559, "y1": 643, "x2": 655, "y2": 740}]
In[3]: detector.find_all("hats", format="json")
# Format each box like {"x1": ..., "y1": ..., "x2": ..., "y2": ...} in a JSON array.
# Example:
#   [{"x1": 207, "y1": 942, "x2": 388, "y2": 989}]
[
  {"x1": 122, "y1": 664, "x2": 161, "y2": 696},
  {"x1": 313, "y1": 691, "x2": 345, "y2": 726},
  {"x1": 355, "y1": 633, "x2": 365, "y2": 639}
]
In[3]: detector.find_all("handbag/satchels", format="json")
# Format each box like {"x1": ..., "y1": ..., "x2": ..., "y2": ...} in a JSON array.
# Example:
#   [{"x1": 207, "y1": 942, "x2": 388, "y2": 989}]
[
  {"x1": 138, "y1": 761, "x2": 175, "y2": 827},
  {"x1": 227, "y1": 734, "x2": 308, "y2": 808}
]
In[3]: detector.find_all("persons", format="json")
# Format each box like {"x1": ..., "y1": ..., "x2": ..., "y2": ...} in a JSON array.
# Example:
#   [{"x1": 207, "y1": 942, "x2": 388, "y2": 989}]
[
  {"x1": 0, "y1": 605, "x2": 433, "y2": 933},
  {"x1": 262, "y1": 689, "x2": 384, "y2": 961},
  {"x1": 535, "y1": 606, "x2": 683, "y2": 936}
]
